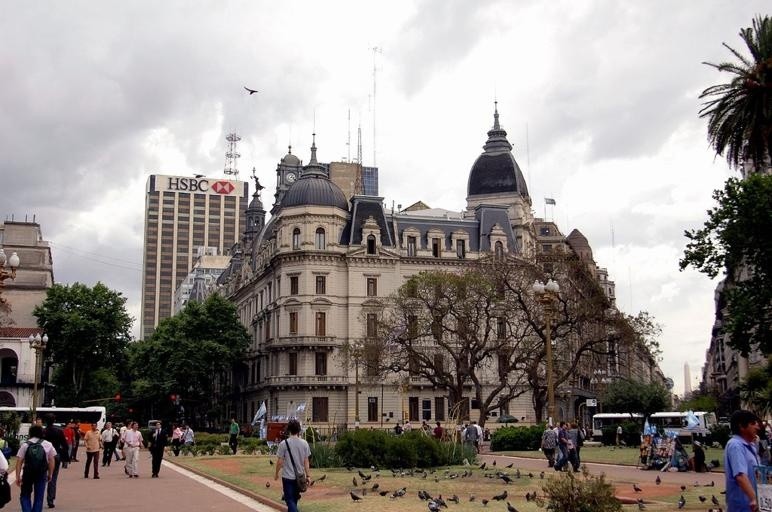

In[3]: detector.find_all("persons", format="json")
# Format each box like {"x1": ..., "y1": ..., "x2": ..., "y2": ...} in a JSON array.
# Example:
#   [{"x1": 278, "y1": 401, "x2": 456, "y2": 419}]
[
  {"x1": 541, "y1": 421, "x2": 589, "y2": 472},
  {"x1": 394, "y1": 421, "x2": 489, "y2": 454},
  {"x1": 616, "y1": 424, "x2": 623, "y2": 446},
  {"x1": 690, "y1": 440, "x2": 707, "y2": 472},
  {"x1": 229, "y1": 418, "x2": 240, "y2": 454},
  {"x1": 0, "y1": 413, "x2": 195, "y2": 512},
  {"x1": 724, "y1": 409, "x2": 760, "y2": 511},
  {"x1": 275, "y1": 420, "x2": 312, "y2": 512}
]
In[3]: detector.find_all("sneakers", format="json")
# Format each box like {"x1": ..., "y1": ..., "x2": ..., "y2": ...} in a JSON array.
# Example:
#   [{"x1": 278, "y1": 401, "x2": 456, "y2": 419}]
[
  {"x1": 101, "y1": 457, "x2": 126, "y2": 467},
  {"x1": 83, "y1": 474, "x2": 99, "y2": 480},
  {"x1": 122, "y1": 470, "x2": 159, "y2": 479},
  {"x1": 548, "y1": 465, "x2": 581, "y2": 473},
  {"x1": 47, "y1": 499, "x2": 55, "y2": 509}
]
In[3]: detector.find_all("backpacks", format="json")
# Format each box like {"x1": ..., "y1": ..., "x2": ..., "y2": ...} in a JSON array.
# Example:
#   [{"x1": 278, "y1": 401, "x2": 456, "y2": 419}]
[{"x1": 23, "y1": 438, "x2": 49, "y2": 481}]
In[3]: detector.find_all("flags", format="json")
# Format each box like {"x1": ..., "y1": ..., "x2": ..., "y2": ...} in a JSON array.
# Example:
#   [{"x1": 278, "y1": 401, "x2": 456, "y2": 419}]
[{"x1": 250, "y1": 401, "x2": 267, "y2": 426}]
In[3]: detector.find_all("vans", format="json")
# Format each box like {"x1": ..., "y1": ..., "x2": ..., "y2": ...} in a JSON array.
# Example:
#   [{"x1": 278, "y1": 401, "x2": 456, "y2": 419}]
[{"x1": 147, "y1": 420, "x2": 162, "y2": 432}]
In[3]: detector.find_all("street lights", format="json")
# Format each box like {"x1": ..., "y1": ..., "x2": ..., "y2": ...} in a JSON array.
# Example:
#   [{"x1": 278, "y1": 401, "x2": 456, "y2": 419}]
[
  {"x1": 28, "y1": 332, "x2": 48, "y2": 427},
  {"x1": 532, "y1": 278, "x2": 563, "y2": 429},
  {"x1": 0, "y1": 249, "x2": 20, "y2": 294},
  {"x1": 379, "y1": 372, "x2": 386, "y2": 430},
  {"x1": 590, "y1": 367, "x2": 613, "y2": 412},
  {"x1": 353, "y1": 337, "x2": 367, "y2": 431},
  {"x1": 560, "y1": 389, "x2": 572, "y2": 423},
  {"x1": 500, "y1": 392, "x2": 511, "y2": 415}
]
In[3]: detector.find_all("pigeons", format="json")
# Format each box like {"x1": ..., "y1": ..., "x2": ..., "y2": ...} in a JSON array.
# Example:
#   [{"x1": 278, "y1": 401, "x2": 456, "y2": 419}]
[
  {"x1": 298, "y1": 494, "x2": 301, "y2": 500},
  {"x1": 656, "y1": 475, "x2": 661, "y2": 486},
  {"x1": 243, "y1": 86, "x2": 258, "y2": 95},
  {"x1": 632, "y1": 483, "x2": 642, "y2": 492},
  {"x1": 678, "y1": 481, "x2": 726, "y2": 512},
  {"x1": 318, "y1": 474, "x2": 326, "y2": 481},
  {"x1": 265, "y1": 481, "x2": 271, "y2": 488},
  {"x1": 638, "y1": 502, "x2": 646, "y2": 510},
  {"x1": 269, "y1": 459, "x2": 274, "y2": 465},
  {"x1": 637, "y1": 498, "x2": 644, "y2": 503},
  {"x1": 310, "y1": 480, "x2": 315, "y2": 487},
  {"x1": 345, "y1": 460, "x2": 549, "y2": 512},
  {"x1": 282, "y1": 494, "x2": 285, "y2": 501}
]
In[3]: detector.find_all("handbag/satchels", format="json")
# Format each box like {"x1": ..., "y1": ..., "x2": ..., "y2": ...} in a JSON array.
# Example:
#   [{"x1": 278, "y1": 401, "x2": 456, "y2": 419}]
[{"x1": 296, "y1": 473, "x2": 307, "y2": 492}]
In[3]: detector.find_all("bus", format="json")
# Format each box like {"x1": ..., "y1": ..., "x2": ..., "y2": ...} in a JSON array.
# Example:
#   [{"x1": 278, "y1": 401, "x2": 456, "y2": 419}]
[
  {"x1": 693, "y1": 412, "x2": 718, "y2": 433},
  {"x1": 592, "y1": 412, "x2": 700, "y2": 440},
  {"x1": 0, "y1": 406, "x2": 106, "y2": 450}
]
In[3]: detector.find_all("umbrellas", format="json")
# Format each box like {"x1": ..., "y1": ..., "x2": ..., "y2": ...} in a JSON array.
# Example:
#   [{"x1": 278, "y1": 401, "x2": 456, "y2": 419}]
[{"x1": 495, "y1": 414, "x2": 519, "y2": 428}]
